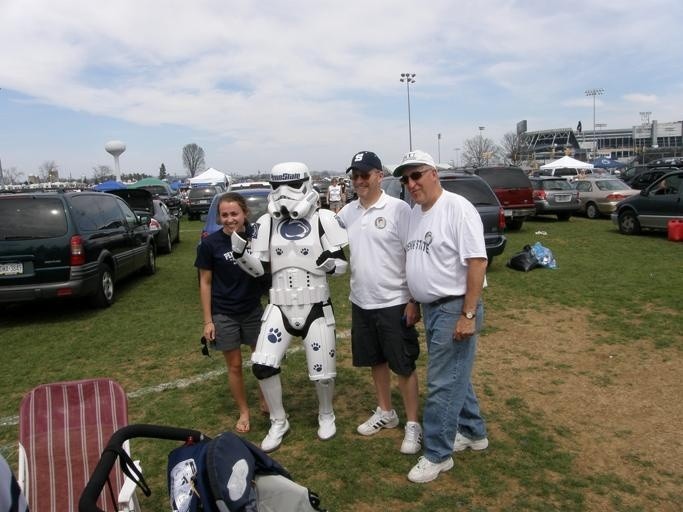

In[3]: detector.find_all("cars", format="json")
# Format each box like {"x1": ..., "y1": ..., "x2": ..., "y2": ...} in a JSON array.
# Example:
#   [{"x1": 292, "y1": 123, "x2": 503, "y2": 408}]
[
  {"x1": 571, "y1": 177, "x2": 640, "y2": 219},
  {"x1": 379, "y1": 175, "x2": 506, "y2": 268},
  {"x1": 134, "y1": 199, "x2": 178, "y2": 252},
  {"x1": 621, "y1": 163, "x2": 679, "y2": 188},
  {"x1": 186, "y1": 182, "x2": 290, "y2": 281},
  {"x1": 531, "y1": 175, "x2": 583, "y2": 222},
  {"x1": 310, "y1": 173, "x2": 354, "y2": 210}
]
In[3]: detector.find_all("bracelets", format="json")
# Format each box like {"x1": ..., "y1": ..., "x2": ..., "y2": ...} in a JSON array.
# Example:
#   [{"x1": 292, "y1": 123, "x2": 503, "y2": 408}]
[
  {"x1": 203, "y1": 320, "x2": 214, "y2": 327},
  {"x1": 409, "y1": 298, "x2": 420, "y2": 304}
]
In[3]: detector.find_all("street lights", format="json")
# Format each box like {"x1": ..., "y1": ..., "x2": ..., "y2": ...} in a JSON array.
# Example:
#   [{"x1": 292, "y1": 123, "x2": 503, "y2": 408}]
[
  {"x1": 399, "y1": 71, "x2": 417, "y2": 153},
  {"x1": 583, "y1": 87, "x2": 604, "y2": 164}
]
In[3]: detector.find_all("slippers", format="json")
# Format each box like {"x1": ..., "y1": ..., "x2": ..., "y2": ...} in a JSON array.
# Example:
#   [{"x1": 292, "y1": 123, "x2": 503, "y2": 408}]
[{"x1": 234, "y1": 416, "x2": 249, "y2": 432}]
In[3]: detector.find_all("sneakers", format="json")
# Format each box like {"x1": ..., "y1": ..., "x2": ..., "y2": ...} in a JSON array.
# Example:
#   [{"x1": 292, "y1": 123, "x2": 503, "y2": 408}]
[
  {"x1": 399, "y1": 420, "x2": 424, "y2": 453},
  {"x1": 452, "y1": 430, "x2": 489, "y2": 451},
  {"x1": 357, "y1": 408, "x2": 399, "y2": 435},
  {"x1": 408, "y1": 457, "x2": 454, "y2": 484}
]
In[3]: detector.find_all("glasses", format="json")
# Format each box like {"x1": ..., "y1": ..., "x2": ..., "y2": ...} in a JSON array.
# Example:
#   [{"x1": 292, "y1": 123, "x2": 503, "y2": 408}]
[
  {"x1": 400, "y1": 168, "x2": 432, "y2": 180},
  {"x1": 200, "y1": 335, "x2": 216, "y2": 362},
  {"x1": 348, "y1": 174, "x2": 371, "y2": 179}
]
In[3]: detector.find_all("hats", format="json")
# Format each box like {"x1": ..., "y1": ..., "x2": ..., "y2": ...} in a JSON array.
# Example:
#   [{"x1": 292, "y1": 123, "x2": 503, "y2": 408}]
[
  {"x1": 391, "y1": 151, "x2": 437, "y2": 177},
  {"x1": 345, "y1": 152, "x2": 381, "y2": 173}
]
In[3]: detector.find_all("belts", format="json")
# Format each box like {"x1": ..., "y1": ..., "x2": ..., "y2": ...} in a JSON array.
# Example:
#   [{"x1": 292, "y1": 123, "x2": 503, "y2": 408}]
[{"x1": 428, "y1": 295, "x2": 468, "y2": 307}]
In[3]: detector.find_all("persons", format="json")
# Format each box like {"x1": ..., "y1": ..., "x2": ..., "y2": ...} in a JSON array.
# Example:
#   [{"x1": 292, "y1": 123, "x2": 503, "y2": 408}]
[
  {"x1": 396, "y1": 148, "x2": 493, "y2": 483},
  {"x1": 330, "y1": 151, "x2": 425, "y2": 456},
  {"x1": 230, "y1": 161, "x2": 349, "y2": 455},
  {"x1": 198, "y1": 192, "x2": 276, "y2": 433},
  {"x1": 326, "y1": 176, "x2": 346, "y2": 213}
]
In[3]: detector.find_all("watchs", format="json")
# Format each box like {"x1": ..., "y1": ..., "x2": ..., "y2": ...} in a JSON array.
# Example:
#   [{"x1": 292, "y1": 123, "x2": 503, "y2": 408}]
[{"x1": 459, "y1": 310, "x2": 478, "y2": 320}]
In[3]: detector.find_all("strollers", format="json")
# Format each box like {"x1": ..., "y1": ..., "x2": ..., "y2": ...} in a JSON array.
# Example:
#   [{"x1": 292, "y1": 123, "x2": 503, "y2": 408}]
[{"x1": 78, "y1": 425, "x2": 330, "y2": 512}]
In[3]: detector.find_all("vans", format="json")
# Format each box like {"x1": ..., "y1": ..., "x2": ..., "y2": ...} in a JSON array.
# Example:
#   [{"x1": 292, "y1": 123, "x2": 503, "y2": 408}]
[
  {"x1": 472, "y1": 164, "x2": 534, "y2": 231},
  {"x1": 0, "y1": 191, "x2": 155, "y2": 305},
  {"x1": 610, "y1": 172, "x2": 681, "y2": 236}
]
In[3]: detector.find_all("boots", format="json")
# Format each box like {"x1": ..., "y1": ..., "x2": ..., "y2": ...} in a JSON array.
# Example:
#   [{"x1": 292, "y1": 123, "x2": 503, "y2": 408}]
[
  {"x1": 316, "y1": 379, "x2": 337, "y2": 442},
  {"x1": 259, "y1": 376, "x2": 291, "y2": 453}
]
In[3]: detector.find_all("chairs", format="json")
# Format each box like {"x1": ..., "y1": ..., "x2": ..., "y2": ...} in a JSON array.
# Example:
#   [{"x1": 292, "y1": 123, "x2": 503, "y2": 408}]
[{"x1": 15, "y1": 376, "x2": 142, "y2": 512}]
